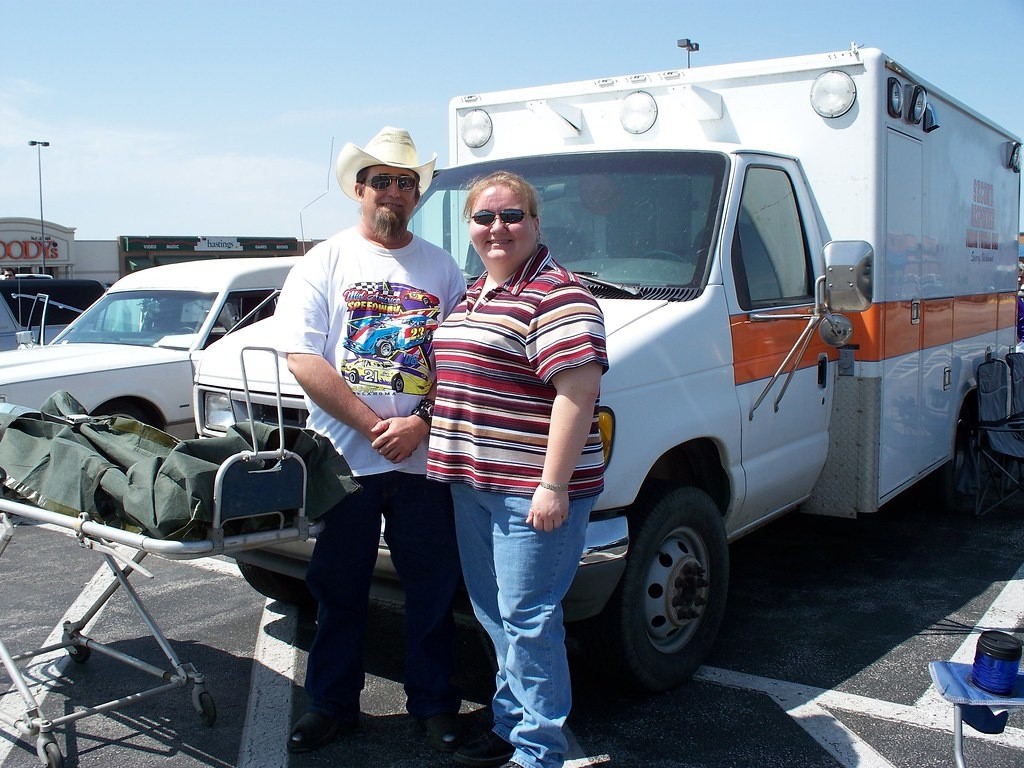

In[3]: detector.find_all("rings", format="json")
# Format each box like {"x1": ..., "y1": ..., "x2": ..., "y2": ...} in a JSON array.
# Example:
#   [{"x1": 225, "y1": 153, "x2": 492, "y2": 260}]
[{"x1": 562, "y1": 516, "x2": 567, "y2": 519}]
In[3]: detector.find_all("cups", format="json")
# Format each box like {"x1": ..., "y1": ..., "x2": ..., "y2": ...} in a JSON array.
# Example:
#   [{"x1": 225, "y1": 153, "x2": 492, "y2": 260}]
[{"x1": 971, "y1": 630, "x2": 1024, "y2": 695}]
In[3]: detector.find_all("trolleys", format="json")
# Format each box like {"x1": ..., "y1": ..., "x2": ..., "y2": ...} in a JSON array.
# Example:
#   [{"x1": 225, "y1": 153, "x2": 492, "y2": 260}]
[{"x1": 0, "y1": 345, "x2": 325, "y2": 767}]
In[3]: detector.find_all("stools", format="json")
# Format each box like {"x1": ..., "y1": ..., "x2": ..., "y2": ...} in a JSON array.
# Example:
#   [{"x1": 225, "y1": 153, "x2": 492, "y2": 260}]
[{"x1": 929, "y1": 662, "x2": 1024, "y2": 768}]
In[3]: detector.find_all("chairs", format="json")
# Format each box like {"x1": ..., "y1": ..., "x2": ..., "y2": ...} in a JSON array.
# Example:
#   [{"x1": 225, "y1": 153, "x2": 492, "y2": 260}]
[{"x1": 540, "y1": 227, "x2": 587, "y2": 265}]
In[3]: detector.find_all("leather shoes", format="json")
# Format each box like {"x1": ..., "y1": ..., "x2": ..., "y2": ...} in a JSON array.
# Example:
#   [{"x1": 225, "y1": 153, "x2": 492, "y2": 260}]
[
  {"x1": 286, "y1": 711, "x2": 358, "y2": 752},
  {"x1": 452, "y1": 730, "x2": 514, "y2": 767},
  {"x1": 417, "y1": 712, "x2": 461, "y2": 752}
]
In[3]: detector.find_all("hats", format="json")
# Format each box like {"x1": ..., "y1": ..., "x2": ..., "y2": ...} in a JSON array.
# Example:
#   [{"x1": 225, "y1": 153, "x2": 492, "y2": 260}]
[{"x1": 335, "y1": 125, "x2": 437, "y2": 202}]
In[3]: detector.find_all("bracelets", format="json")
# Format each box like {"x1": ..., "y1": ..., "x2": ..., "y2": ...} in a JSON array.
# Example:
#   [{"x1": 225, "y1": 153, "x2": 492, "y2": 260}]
[
  {"x1": 413, "y1": 397, "x2": 435, "y2": 426},
  {"x1": 540, "y1": 481, "x2": 569, "y2": 491}
]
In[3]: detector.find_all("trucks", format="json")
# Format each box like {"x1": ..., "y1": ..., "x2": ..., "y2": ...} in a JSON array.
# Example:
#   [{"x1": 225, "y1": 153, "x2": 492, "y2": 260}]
[{"x1": 185, "y1": 47, "x2": 1023, "y2": 698}]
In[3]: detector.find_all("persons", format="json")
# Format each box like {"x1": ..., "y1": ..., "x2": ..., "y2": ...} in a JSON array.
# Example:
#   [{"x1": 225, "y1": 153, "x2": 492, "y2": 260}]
[
  {"x1": 425, "y1": 171, "x2": 612, "y2": 768},
  {"x1": 270, "y1": 124, "x2": 469, "y2": 754},
  {"x1": 4, "y1": 267, "x2": 15, "y2": 280}
]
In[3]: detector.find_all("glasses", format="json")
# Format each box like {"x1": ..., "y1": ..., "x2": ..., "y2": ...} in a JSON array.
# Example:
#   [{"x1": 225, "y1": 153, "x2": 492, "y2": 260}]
[
  {"x1": 471, "y1": 208, "x2": 530, "y2": 225},
  {"x1": 364, "y1": 174, "x2": 418, "y2": 192}
]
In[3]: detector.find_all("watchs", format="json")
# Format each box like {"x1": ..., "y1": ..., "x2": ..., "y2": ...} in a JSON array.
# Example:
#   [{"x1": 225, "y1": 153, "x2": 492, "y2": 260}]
[{"x1": 417, "y1": 402, "x2": 435, "y2": 418}]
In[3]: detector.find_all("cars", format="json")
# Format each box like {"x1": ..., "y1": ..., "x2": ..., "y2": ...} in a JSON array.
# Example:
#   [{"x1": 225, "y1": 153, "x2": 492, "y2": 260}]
[{"x1": 1, "y1": 257, "x2": 307, "y2": 459}]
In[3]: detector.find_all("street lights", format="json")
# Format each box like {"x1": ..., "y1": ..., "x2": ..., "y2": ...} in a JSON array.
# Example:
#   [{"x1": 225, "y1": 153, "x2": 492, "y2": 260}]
[{"x1": 28, "y1": 140, "x2": 50, "y2": 275}]
